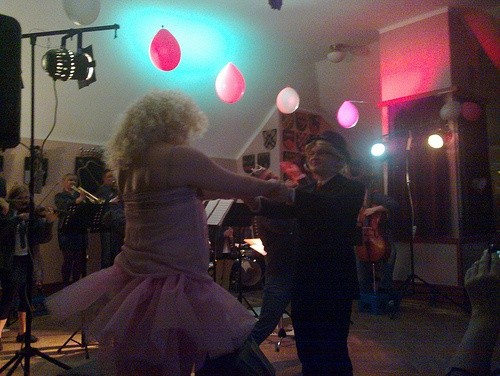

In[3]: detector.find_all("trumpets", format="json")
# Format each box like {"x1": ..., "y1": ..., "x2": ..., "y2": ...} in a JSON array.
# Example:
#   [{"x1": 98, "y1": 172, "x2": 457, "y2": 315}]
[{"x1": 72, "y1": 185, "x2": 104, "y2": 204}]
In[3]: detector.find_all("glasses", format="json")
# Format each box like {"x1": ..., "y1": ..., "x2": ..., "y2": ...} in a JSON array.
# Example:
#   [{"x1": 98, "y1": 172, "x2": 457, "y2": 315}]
[{"x1": 307, "y1": 149, "x2": 336, "y2": 158}]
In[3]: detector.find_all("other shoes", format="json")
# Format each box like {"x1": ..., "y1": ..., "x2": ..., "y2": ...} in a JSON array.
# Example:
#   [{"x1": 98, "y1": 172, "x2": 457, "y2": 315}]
[
  {"x1": 16, "y1": 331, "x2": 38, "y2": 343},
  {"x1": 0, "y1": 338, "x2": 3, "y2": 350}
]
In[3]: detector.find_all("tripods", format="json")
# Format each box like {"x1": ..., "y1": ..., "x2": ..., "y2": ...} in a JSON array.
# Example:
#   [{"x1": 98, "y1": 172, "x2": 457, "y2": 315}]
[
  {"x1": 56, "y1": 202, "x2": 99, "y2": 359},
  {"x1": 223, "y1": 224, "x2": 258, "y2": 316},
  {"x1": 388, "y1": 151, "x2": 473, "y2": 319}
]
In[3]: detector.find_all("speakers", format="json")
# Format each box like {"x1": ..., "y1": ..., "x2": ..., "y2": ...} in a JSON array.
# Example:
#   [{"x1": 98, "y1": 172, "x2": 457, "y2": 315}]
[{"x1": 0, "y1": 14, "x2": 20, "y2": 150}]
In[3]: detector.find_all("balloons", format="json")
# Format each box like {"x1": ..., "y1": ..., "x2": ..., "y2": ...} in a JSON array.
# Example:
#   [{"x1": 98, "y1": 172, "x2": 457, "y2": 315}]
[
  {"x1": 150, "y1": 29, "x2": 181, "y2": 71},
  {"x1": 215, "y1": 62, "x2": 246, "y2": 104},
  {"x1": 336, "y1": 100, "x2": 359, "y2": 128},
  {"x1": 461, "y1": 101, "x2": 481, "y2": 121},
  {"x1": 276, "y1": 86, "x2": 300, "y2": 114},
  {"x1": 61, "y1": 0, "x2": 101, "y2": 26},
  {"x1": 439, "y1": 101, "x2": 460, "y2": 121}
]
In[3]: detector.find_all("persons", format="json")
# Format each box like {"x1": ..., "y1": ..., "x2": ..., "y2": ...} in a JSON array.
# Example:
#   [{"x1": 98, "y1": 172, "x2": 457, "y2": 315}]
[
  {"x1": 45, "y1": 89, "x2": 292, "y2": 376},
  {"x1": 54, "y1": 173, "x2": 93, "y2": 287},
  {"x1": 214, "y1": 225, "x2": 238, "y2": 291},
  {"x1": 242, "y1": 131, "x2": 365, "y2": 376},
  {"x1": 0, "y1": 184, "x2": 54, "y2": 352},
  {"x1": 365, "y1": 189, "x2": 405, "y2": 318},
  {"x1": 445, "y1": 249, "x2": 500, "y2": 376},
  {"x1": 251, "y1": 215, "x2": 294, "y2": 345},
  {"x1": 95, "y1": 168, "x2": 126, "y2": 270}
]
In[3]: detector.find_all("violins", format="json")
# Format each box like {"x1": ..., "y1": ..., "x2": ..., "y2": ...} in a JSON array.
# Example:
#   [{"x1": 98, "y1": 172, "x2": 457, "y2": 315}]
[{"x1": 18, "y1": 205, "x2": 72, "y2": 218}]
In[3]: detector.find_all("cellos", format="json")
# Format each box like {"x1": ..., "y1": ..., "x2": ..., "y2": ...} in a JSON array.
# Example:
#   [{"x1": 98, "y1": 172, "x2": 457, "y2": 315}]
[{"x1": 356, "y1": 169, "x2": 391, "y2": 296}]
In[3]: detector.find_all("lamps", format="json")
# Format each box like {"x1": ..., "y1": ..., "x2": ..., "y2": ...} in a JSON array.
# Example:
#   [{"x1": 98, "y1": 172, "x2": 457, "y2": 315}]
[
  {"x1": 327, "y1": 44, "x2": 344, "y2": 63},
  {"x1": 42, "y1": 31, "x2": 97, "y2": 89}
]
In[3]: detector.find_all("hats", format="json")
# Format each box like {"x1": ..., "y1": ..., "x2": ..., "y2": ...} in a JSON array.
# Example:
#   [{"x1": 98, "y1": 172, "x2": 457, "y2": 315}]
[{"x1": 307, "y1": 130, "x2": 351, "y2": 165}]
